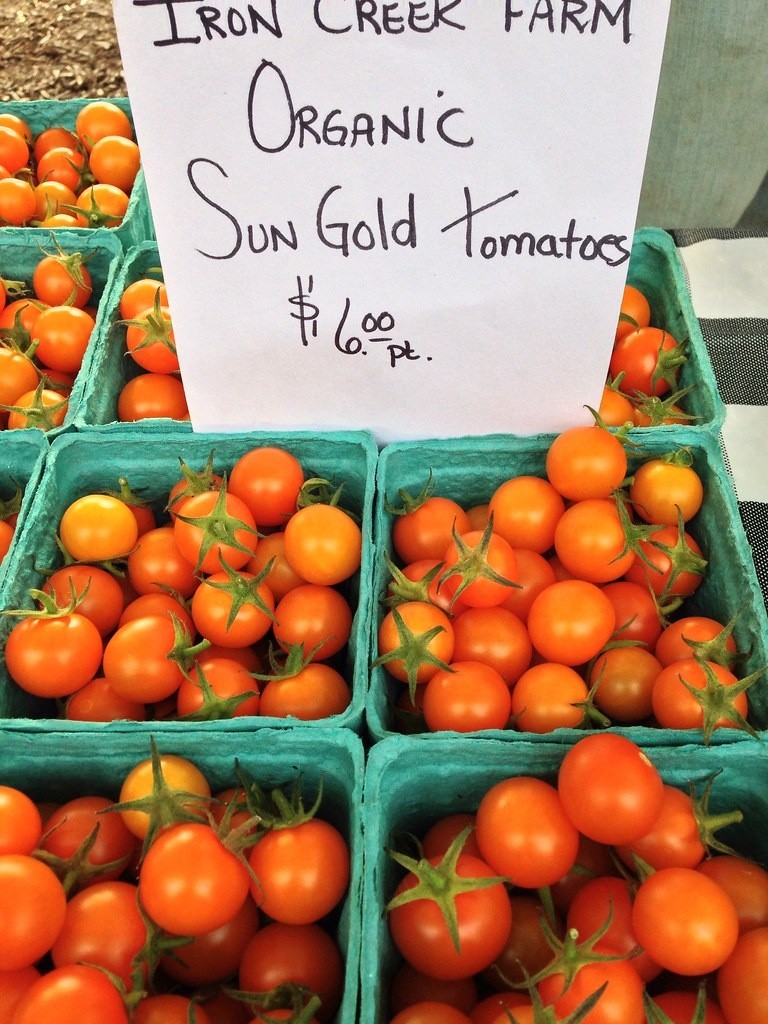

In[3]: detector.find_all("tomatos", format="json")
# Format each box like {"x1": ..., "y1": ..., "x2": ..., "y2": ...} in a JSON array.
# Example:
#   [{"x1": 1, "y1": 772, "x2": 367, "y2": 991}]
[{"x1": 0, "y1": 102, "x2": 767, "y2": 1024}]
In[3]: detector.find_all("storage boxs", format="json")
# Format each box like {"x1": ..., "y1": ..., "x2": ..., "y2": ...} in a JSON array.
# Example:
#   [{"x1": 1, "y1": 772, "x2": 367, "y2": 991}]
[{"x1": 0, "y1": 98, "x2": 768, "y2": 1024}]
